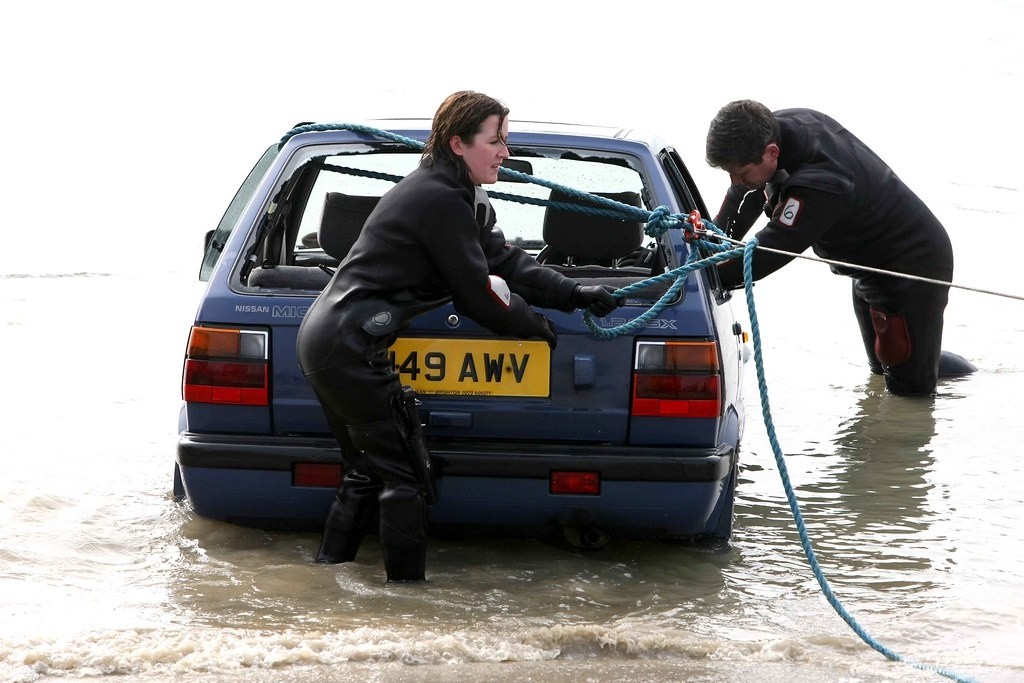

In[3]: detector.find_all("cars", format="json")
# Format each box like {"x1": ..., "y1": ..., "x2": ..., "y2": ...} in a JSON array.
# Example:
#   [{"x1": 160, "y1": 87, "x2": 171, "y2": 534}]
[{"x1": 173, "y1": 120, "x2": 746, "y2": 552}]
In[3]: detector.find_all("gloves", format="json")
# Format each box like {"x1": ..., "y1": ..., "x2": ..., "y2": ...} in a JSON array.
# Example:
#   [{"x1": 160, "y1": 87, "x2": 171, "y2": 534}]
[
  {"x1": 541, "y1": 315, "x2": 558, "y2": 350},
  {"x1": 572, "y1": 284, "x2": 627, "y2": 318}
]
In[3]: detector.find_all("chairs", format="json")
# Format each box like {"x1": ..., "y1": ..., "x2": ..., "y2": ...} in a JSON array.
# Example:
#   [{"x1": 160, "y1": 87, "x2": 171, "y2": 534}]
[
  {"x1": 317, "y1": 192, "x2": 382, "y2": 260},
  {"x1": 541, "y1": 188, "x2": 651, "y2": 278}
]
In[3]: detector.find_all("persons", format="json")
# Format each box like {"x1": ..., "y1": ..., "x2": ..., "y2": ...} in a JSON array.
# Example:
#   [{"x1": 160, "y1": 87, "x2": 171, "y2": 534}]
[
  {"x1": 707, "y1": 99, "x2": 977, "y2": 398},
  {"x1": 296, "y1": 91, "x2": 626, "y2": 584}
]
